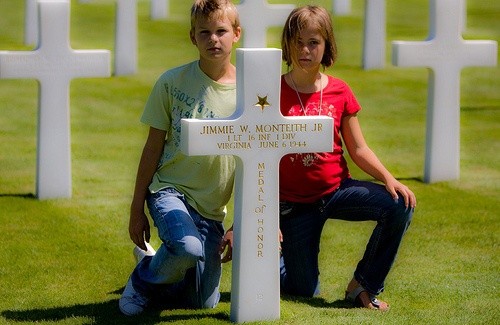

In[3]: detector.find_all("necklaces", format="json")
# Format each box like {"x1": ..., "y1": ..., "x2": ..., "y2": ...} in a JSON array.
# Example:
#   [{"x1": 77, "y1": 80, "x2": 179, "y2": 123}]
[{"x1": 287, "y1": 70, "x2": 324, "y2": 117}]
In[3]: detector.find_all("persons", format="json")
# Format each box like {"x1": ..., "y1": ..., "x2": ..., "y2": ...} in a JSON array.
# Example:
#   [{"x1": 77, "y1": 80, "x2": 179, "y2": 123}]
[
  {"x1": 118, "y1": 0, "x2": 244, "y2": 322},
  {"x1": 278, "y1": 5, "x2": 418, "y2": 313}
]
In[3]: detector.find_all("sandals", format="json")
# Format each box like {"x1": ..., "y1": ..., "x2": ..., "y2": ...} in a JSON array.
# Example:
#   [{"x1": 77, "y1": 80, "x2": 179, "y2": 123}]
[{"x1": 344, "y1": 286, "x2": 390, "y2": 310}]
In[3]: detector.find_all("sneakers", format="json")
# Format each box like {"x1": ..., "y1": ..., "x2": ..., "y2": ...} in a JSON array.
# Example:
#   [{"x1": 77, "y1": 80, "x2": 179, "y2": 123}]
[
  {"x1": 116, "y1": 269, "x2": 146, "y2": 315},
  {"x1": 133, "y1": 241, "x2": 157, "y2": 266}
]
